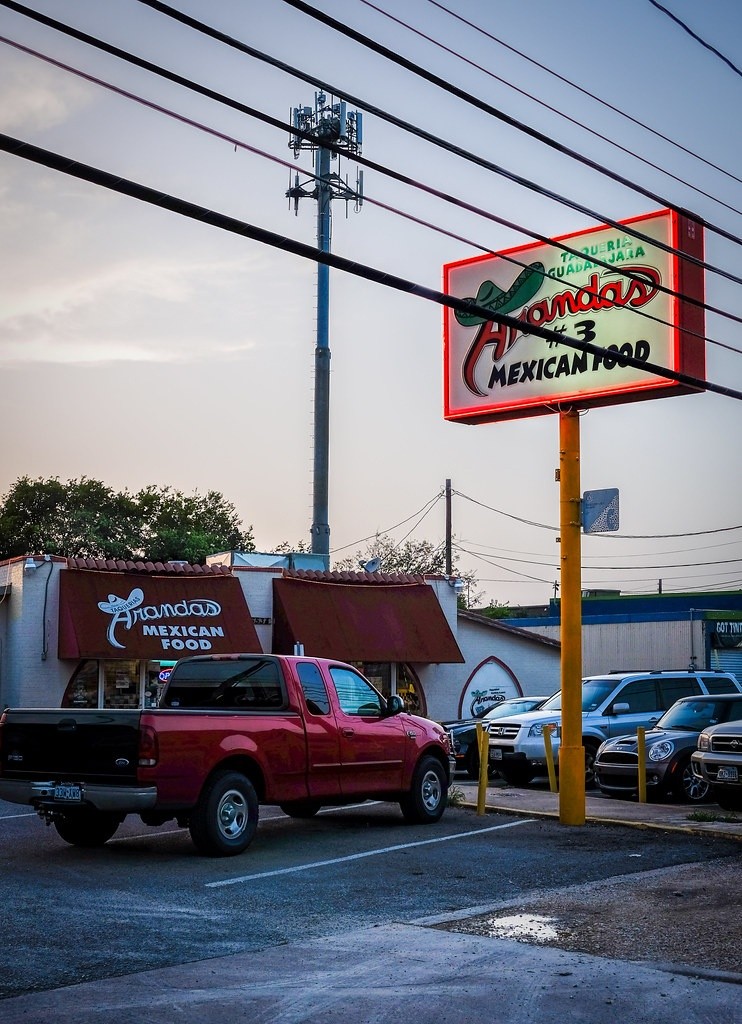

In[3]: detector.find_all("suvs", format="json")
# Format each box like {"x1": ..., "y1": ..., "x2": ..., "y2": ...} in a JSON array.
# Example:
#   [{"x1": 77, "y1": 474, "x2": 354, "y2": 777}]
[
  {"x1": 591, "y1": 692, "x2": 742, "y2": 803},
  {"x1": 483, "y1": 668, "x2": 742, "y2": 794}
]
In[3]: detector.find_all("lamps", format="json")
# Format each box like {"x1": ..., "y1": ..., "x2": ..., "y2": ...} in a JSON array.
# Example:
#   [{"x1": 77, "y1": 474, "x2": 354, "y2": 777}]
[
  {"x1": 445, "y1": 575, "x2": 464, "y2": 595},
  {"x1": 24, "y1": 557, "x2": 36, "y2": 575}
]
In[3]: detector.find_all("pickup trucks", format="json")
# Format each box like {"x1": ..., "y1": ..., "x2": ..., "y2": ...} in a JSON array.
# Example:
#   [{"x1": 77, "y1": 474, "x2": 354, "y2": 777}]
[{"x1": 0, "y1": 652, "x2": 459, "y2": 858}]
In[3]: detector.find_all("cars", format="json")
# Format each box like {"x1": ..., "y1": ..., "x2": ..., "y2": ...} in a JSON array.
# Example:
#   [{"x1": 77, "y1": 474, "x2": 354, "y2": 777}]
[
  {"x1": 691, "y1": 719, "x2": 742, "y2": 812},
  {"x1": 436, "y1": 695, "x2": 552, "y2": 781}
]
